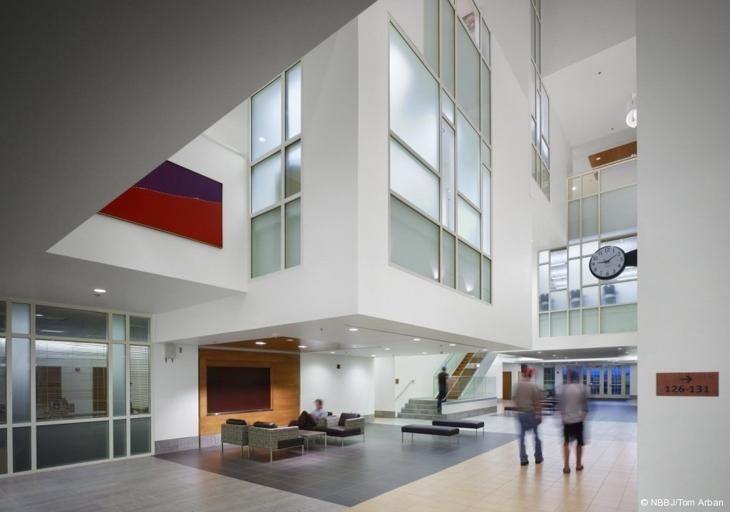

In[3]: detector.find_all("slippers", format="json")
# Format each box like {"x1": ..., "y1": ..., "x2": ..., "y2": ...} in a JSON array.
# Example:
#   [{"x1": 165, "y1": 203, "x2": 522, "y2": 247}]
[
  {"x1": 576, "y1": 465, "x2": 583, "y2": 470},
  {"x1": 564, "y1": 467, "x2": 570, "y2": 473}
]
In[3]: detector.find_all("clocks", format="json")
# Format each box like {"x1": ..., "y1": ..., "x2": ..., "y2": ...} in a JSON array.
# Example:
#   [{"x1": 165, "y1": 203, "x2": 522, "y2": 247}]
[{"x1": 587, "y1": 244, "x2": 638, "y2": 281}]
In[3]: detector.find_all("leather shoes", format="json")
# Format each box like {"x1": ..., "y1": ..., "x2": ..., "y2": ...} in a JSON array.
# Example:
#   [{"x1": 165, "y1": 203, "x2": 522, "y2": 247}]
[
  {"x1": 521, "y1": 462, "x2": 528, "y2": 465},
  {"x1": 536, "y1": 460, "x2": 541, "y2": 463}
]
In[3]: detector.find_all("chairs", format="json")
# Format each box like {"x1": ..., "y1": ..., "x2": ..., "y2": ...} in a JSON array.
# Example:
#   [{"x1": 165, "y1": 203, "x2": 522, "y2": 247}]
[{"x1": 218, "y1": 409, "x2": 367, "y2": 463}]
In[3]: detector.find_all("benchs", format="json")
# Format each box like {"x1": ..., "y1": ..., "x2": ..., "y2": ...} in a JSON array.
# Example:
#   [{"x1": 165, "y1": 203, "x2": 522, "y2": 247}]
[
  {"x1": 400, "y1": 422, "x2": 462, "y2": 448},
  {"x1": 432, "y1": 416, "x2": 486, "y2": 442}
]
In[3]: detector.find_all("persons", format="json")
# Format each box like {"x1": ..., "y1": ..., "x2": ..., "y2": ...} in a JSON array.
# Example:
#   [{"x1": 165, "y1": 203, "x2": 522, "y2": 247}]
[
  {"x1": 558, "y1": 369, "x2": 590, "y2": 472},
  {"x1": 290, "y1": 398, "x2": 328, "y2": 429},
  {"x1": 437, "y1": 367, "x2": 449, "y2": 411},
  {"x1": 514, "y1": 367, "x2": 543, "y2": 465}
]
